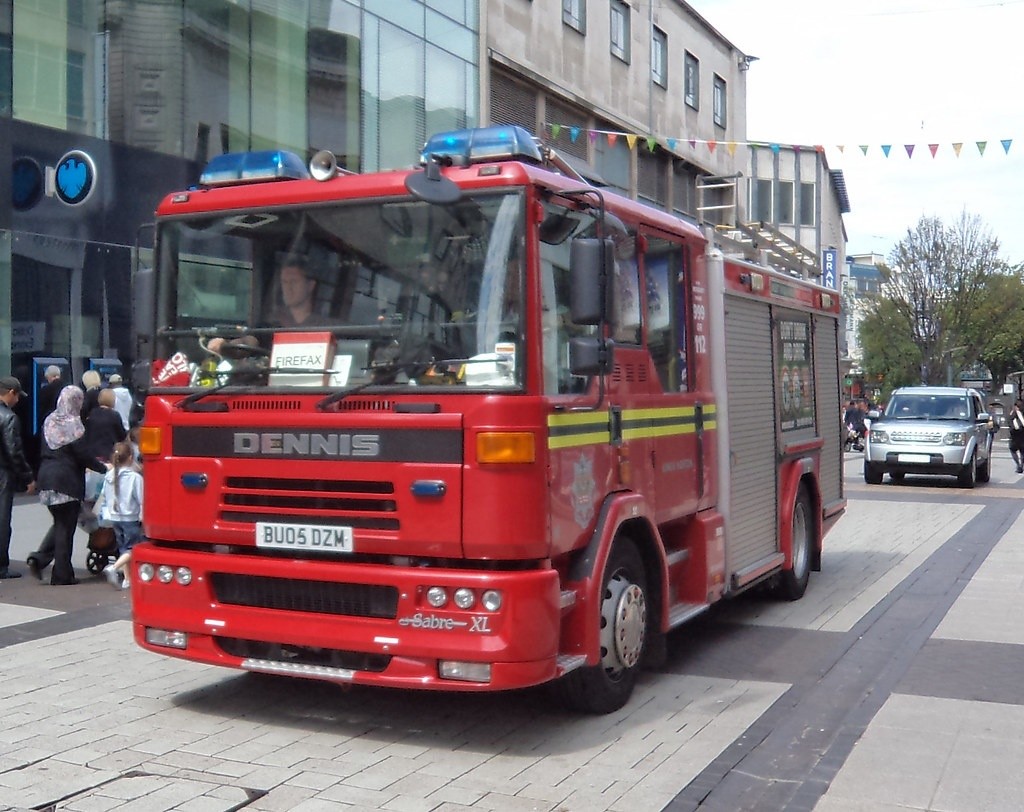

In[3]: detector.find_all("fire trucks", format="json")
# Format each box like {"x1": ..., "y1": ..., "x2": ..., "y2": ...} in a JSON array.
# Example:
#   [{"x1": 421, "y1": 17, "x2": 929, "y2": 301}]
[{"x1": 130, "y1": 122, "x2": 852, "y2": 719}]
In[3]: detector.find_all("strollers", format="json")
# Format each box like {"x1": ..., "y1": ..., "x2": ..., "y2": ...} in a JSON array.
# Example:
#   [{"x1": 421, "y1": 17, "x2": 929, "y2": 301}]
[{"x1": 77, "y1": 454, "x2": 125, "y2": 578}]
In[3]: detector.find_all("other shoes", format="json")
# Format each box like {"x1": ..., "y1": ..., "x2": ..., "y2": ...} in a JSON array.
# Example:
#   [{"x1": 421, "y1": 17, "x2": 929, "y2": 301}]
[
  {"x1": 121, "y1": 579, "x2": 130, "y2": 589},
  {"x1": 104, "y1": 564, "x2": 120, "y2": 589},
  {"x1": 28, "y1": 558, "x2": 42, "y2": 580},
  {"x1": 51, "y1": 577, "x2": 81, "y2": 586},
  {"x1": 0, "y1": 571, "x2": 22, "y2": 579},
  {"x1": 1015, "y1": 467, "x2": 1023, "y2": 473}
]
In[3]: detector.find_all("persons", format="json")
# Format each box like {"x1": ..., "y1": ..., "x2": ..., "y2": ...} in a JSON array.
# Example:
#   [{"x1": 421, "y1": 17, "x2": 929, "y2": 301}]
[
  {"x1": 79, "y1": 371, "x2": 142, "y2": 473},
  {"x1": 1008, "y1": 401, "x2": 1024, "y2": 472},
  {"x1": 203, "y1": 258, "x2": 350, "y2": 353},
  {"x1": 0, "y1": 377, "x2": 37, "y2": 579},
  {"x1": 842, "y1": 394, "x2": 885, "y2": 449},
  {"x1": 27, "y1": 385, "x2": 114, "y2": 586},
  {"x1": 100, "y1": 441, "x2": 144, "y2": 589},
  {"x1": 496, "y1": 261, "x2": 578, "y2": 337},
  {"x1": 36, "y1": 365, "x2": 73, "y2": 465}
]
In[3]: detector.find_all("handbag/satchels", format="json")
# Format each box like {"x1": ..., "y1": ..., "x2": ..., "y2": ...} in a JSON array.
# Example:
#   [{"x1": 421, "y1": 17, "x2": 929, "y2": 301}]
[{"x1": 76, "y1": 507, "x2": 99, "y2": 534}]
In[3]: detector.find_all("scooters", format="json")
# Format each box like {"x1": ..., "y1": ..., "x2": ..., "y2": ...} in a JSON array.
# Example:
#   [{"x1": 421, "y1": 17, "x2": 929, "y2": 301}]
[{"x1": 842, "y1": 421, "x2": 865, "y2": 452}]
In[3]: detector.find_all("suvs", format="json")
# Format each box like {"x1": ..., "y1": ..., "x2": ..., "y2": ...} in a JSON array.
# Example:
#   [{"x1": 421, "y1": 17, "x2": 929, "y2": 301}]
[{"x1": 863, "y1": 384, "x2": 995, "y2": 490}]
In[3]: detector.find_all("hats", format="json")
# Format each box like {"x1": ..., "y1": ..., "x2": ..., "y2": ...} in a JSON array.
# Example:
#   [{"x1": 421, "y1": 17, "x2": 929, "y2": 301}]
[
  {"x1": 109, "y1": 374, "x2": 122, "y2": 382},
  {"x1": 0, "y1": 377, "x2": 28, "y2": 397}
]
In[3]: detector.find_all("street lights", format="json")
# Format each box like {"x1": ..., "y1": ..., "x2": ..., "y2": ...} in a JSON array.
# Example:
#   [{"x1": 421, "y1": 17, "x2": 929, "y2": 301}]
[{"x1": 911, "y1": 317, "x2": 937, "y2": 389}]
